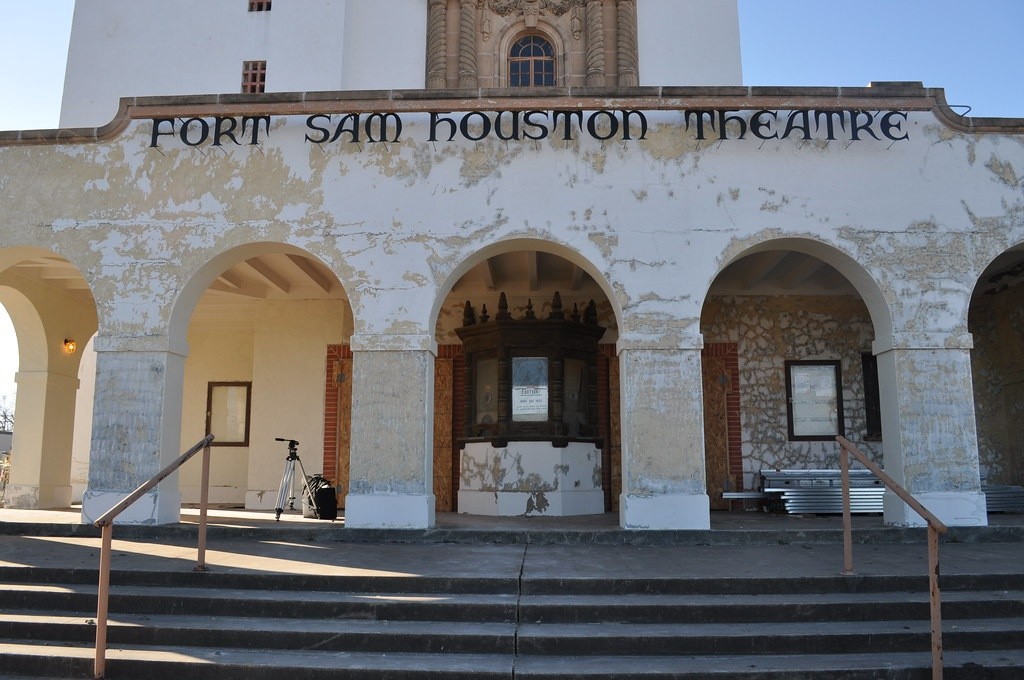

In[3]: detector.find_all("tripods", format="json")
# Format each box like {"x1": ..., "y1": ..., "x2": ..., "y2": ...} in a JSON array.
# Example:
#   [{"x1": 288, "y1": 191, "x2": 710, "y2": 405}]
[{"x1": 274, "y1": 438, "x2": 320, "y2": 521}]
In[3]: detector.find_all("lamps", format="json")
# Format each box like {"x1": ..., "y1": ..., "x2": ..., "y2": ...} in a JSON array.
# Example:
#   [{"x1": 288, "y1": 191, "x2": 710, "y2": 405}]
[{"x1": 64, "y1": 338, "x2": 75, "y2": 354}]
